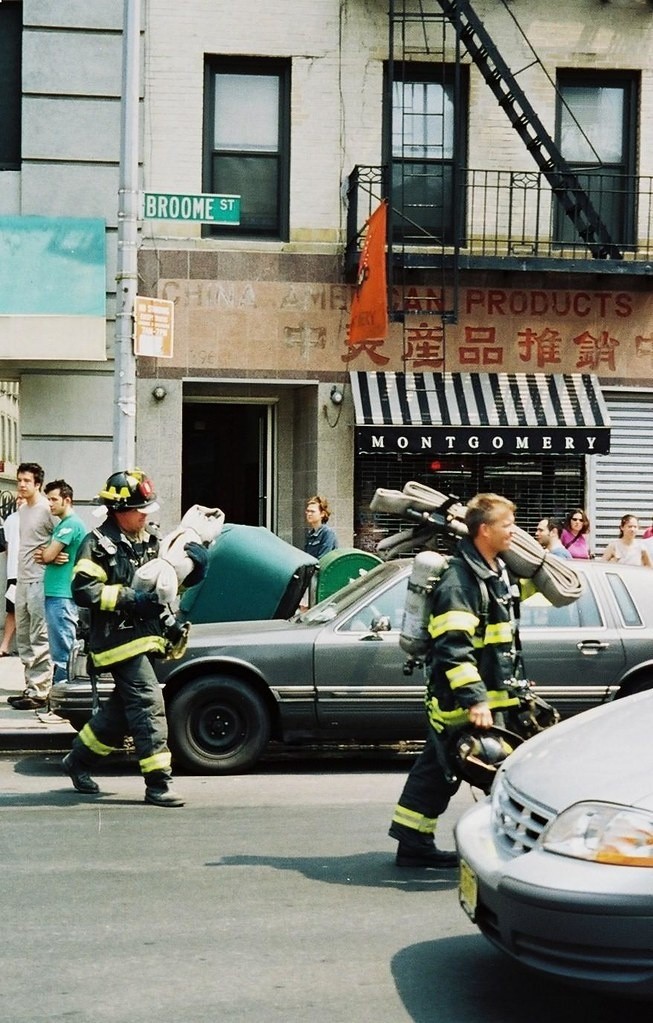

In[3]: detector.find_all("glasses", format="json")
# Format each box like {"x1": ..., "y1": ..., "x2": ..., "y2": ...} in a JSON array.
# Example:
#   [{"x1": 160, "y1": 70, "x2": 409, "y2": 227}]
[
  {"x1": 572, "y1": 517, "x2": 584, "y2": 523},
  {"x1": 305, "y1": 510, "x2": 320, "y2": 514}
]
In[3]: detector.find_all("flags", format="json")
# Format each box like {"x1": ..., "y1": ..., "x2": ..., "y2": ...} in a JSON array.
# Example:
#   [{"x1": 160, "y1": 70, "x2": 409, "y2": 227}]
[{"x1": 348, "y1": 203, "x2": 390, "y2": 346}]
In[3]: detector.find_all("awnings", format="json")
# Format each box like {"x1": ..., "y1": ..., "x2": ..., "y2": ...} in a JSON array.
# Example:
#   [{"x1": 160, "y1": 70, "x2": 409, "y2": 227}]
[
  {"x1": 0, "y1": 217, "x2": 108, "y2": 360},
  {"x1": 349, "y1": 372, "x2": 612, "y2": 456}
]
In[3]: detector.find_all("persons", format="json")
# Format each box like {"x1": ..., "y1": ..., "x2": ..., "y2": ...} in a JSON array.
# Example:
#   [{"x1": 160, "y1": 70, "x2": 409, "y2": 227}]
[
  {"x1": 535, "y1": 508, "x2": 653, "y2": 567},
  {"x1": 299, "y1": 495, "x2": 338, "y2": 612},
  {"x1": 389, "y1": 492, "x2": 544, "y2": 868},
  {"x1": 63, "y1": 468, "x2": 210, "y2": 808},
  {"x1": 0, "y1": 463, "x2": 91, "y2": 724}
]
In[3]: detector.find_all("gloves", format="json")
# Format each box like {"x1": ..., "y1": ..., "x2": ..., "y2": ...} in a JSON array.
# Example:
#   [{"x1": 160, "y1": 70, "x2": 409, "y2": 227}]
[
  {"x1": 183, "y1": 541, "x2": 211, "y2": 587},
  {"x1": 135, "y1": 592, "x2": 166, "y2": 617}
]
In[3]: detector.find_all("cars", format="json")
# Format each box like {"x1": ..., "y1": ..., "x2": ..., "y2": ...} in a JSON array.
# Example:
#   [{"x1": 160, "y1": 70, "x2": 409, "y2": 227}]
[
  {"x1": 452, "y1": 658, "x2": 653, "y2": 1004},
  {"x1": 46, "y1": 553, "x2": 653, "y2": 774}
]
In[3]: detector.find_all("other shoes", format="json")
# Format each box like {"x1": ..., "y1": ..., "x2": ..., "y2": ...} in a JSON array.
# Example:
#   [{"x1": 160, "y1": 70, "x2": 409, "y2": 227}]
[
  {"x1": 61, "y1": 757, "x2": 100, "y2": 794},
  {"x1": 395, "y1": 838, "x2": 461, "y2": 869},
  {"x1": 145, "y1": 788, "x2": 187, "y2": 807},
  {"x1": 9, "y1": 694, "x2": 46, "y2": 710},
  {"x1": 0, "y1": 650, "x2": 10, "y2": 657}
]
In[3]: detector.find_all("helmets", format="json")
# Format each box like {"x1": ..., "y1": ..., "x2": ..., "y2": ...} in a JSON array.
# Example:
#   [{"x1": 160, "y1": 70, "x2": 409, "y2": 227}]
[
  {"x1": 449, "y1": 722, "x2": 525, "y2": 795},
  {"x1": 97, "y1": 470, "x2": 157, "y2": 509}
]
